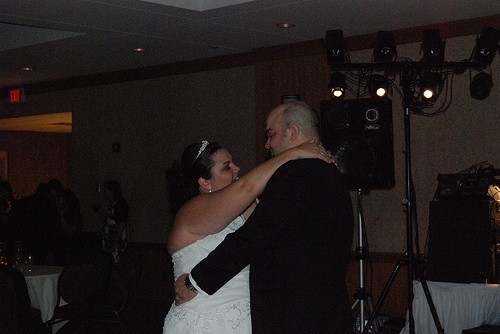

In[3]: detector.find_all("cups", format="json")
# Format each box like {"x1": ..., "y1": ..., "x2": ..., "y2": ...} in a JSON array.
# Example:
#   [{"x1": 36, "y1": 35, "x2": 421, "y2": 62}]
[{"x1": 24, "y1": 256, "x2": 32, "y2": 273}]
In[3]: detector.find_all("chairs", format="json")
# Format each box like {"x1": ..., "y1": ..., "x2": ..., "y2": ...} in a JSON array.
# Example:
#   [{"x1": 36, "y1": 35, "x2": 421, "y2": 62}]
[{"x1": 0, "y1": 232, "x2": 143, "y2": 334}]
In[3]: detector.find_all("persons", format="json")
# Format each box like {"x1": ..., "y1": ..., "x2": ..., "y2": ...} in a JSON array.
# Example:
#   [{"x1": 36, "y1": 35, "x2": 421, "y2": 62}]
[
  {"x1": 0, "y1": 176, "x2": 83, "y2": 264},
  {"x1": 0, "y1": 264, "x2": 46, "y2": 334},
  {"x1": 171, "y1": 99, "x2": 355, "y2": 334},
  {"x1": 162, "y1": 137, "x2": 341, "y2": 334},
  {"x1": 86, "y1": 178, "x2": 132, "y2": 302}
]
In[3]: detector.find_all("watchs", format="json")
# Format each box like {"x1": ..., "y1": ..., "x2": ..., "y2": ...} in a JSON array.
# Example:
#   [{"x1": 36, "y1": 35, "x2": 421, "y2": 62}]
[{"x1": 184, "y1": 274, "x2": 197, "y2": 293}]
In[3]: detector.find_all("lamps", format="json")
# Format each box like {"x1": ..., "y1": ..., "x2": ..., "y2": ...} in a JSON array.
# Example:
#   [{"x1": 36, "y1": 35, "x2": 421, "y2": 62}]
[
  {"x1": 371, "y1": 74, "x2": 390, "y2": 101},
  {"x1": 325, "y1": 45, "x2": 349, "y2": 66},
  {"x1": 371, "y1": 31, "x2": 398, "y2": 64},
  {"x1": 468, "y1": 69, "x2": 494, "y2": 101},
  {"x1": 417, "y1": 29, "x2": 446, "y2": 65},
  {"x1": 468, "y1": 27, "x2": 500, "y2": 67},
  {"x1": 329, "y1": 75, "x2": 348, "y2": 102}
]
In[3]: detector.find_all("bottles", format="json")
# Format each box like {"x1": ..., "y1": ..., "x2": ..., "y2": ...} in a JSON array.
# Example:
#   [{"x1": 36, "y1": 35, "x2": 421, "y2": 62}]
[{"x1": 0, "y1": 240, "x2": 24, "y2": 271}]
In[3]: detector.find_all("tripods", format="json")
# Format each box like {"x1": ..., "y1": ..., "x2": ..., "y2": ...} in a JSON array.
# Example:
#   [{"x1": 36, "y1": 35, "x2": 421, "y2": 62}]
[{"x1": 350, "y1": 96, "x2": 445, "y2": 334}]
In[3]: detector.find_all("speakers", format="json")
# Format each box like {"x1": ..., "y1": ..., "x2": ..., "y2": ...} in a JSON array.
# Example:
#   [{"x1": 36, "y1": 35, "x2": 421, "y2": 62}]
[
  {"x1": 320, "y1": 97, "x2": 395, "y2": 191},
  {"x1": 427, "y1": 196, "x2": 500, "y2": 283}
]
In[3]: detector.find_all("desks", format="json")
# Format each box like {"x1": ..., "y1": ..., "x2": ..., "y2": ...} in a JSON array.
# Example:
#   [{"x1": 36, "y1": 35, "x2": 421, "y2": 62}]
[{"x1": 25, "y1": 266, "x2": 66, "y2": 323}]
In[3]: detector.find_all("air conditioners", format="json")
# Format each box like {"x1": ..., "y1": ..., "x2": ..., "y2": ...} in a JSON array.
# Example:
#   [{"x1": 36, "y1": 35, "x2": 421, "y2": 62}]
[{"x1": 419, "y1": 73, "x2": 440, "y2": 100}]
